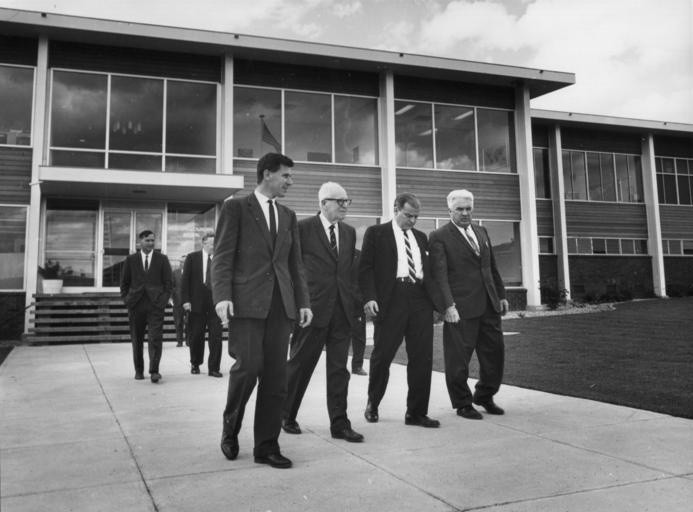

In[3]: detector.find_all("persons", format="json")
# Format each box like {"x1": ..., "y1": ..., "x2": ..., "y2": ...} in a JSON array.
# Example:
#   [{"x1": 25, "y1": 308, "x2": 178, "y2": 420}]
[
  {"x1": 344, "y1": 249, "x2": 369, "y2": 376},
  {"x1": 167, "y1": 255, "x2": 186, "y2": 349},
  {"x1": 182, "y1": 233, "x2": 223, "y2": 377},
  {"x1": 424, "y1": 188, "x2": 510, "y2": 418},
  {"x1": 281, "y1": 183, "x2": 366, "y2": 443},
  {"x1": 361, "y1": 192, "x2": 439, "y2": 428},
  {"x1": 210, "y1": 153, "x2": 312, "y2": 468},
  {"x1": 118, "y1": 227, "x2": 176, "y2": 384}
]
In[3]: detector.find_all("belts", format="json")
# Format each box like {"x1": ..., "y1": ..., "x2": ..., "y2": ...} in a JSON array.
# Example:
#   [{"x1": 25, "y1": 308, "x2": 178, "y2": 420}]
[{"x1": 396, "y1": 277, "x2": 426, "y2": 285}]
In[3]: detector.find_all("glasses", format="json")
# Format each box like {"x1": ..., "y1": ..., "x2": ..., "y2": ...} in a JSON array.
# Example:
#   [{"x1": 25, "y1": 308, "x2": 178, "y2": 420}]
[{"x1": 325, "y1": 199, "x2": 351, "y2": 206}]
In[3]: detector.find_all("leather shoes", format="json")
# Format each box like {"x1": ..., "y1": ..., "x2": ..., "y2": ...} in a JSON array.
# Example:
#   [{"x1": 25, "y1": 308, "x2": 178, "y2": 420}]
[
  {"x1": 331, "y1": 429, "x2": 363, "y2": 442},
  {"x1": 176, "y1": 343, "x2": 190, "y2": 346},
  {"x1": 457, "y1": 398, "x2": 504, "y2": 418},
  {"x1": 405, "y1": 413, "x2": 439, "y2": 428},
  {"x1": 190, "y1": 363, "x2": 223, "y2": 377},
  {"x1": 352, "y1": 368, "x2": 367, "y2": 376},
  {"x1": 365, "y1": 400, "x2": 378, "y2": 422},
  {"x1": 135, "y1": 366, "x2": 161, "y2": 382},
  {"x1": 222, "y1": 416, "x2": 302, "y2": 468}
]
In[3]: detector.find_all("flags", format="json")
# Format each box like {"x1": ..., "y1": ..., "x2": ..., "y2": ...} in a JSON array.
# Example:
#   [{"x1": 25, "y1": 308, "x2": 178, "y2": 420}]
[{"x1": 262, "y1": 119, "x2": 284, "y2": 154}]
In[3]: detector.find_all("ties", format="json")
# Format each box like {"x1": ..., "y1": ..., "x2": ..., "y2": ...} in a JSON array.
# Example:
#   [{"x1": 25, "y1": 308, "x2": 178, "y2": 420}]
[
  {"x1": 402, "y1": 229, "x2": 416, "y2": 286},
  {"x1": 205, "y1": 254, "x2": 212, "y2": 285},
  {"x1": 465, "y1": 227, "x2": 480, "y2": 256},
  {"x1": 267, "y1": 199, "x2": 276, "y2": 249},
  {"x1": 330, "y1": 225, "x2": 338, "y2": 257},
  {"x1": 145, "y1": 256, "x2": 148, "y2": 271}
]
